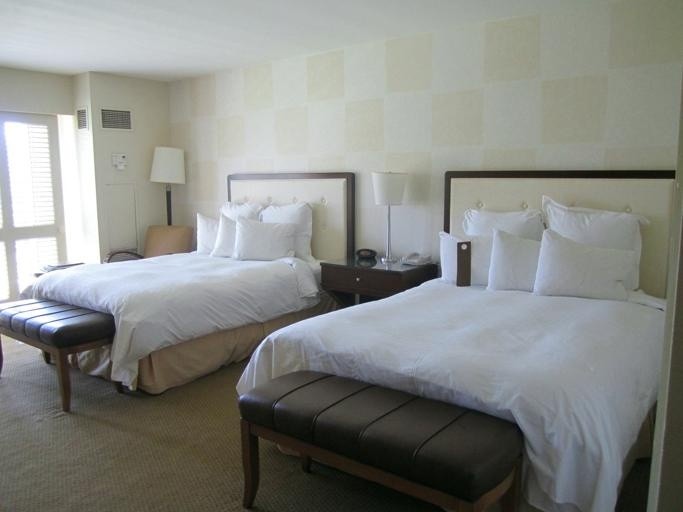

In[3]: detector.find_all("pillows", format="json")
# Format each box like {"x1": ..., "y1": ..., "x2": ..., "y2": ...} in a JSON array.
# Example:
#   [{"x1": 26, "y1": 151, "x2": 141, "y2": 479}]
[
  {"x1": 459, "y1": 204, "x2": 548, "y2": 241},
  {"x1": 215, "y1": 196, "x2": 273, "y2": 223},
  {"x1": 190, "y1": 210, "x2": 221, "y2": 256},
  {"x1": 232, "y1": 213, "x2": 298, "y2": 263},
  {"x1": 532, "y1": 224, "x2": 639, "y2": 302},
  {"x1": 482, "y1": 224, "x2": 540, "y2": 294},
  {"x1": 255, "y1": 202, "x2": 316, "y2": 264},
  {"x1": 541, "y1": 192, "x2": 650, "y2": 292},
  {"x1": 208, "y1": 212, "x2": 238, "y2": 259},
  {"x1": 435, "y1": 231, "x2": 493, "y2": 287}
]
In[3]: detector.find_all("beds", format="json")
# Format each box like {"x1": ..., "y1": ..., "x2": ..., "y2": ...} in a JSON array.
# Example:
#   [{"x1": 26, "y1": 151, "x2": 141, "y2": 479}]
[
  {"x1": 231, "y1": 167, "x2": 676, "y2": 512},
  {"x1": 19, "y1": 171, "x2": 357, "y2": 396}
]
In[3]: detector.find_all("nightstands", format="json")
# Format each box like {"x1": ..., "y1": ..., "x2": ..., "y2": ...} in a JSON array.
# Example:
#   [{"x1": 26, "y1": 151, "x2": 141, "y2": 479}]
[{"x1": 316, "y1": 251, "x2": 439, "y2": 310}]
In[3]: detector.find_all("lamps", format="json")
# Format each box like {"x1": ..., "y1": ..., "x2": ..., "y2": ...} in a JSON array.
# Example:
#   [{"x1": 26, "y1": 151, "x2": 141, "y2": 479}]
[
  {"x1": 367, "y1": 169, "x2": 410, "y2": 264},
  {"x1": 148, "y1": 145, "x2": 187, "y2": 225}
]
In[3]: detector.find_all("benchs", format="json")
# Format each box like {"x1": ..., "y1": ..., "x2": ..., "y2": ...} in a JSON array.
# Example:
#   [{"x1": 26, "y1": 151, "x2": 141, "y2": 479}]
[
  {"x1": 0, "y1": 297, "x2": 124, "y2": 414},
  {"x1": 236, "y1": 367, "x2": 527, "y2": 512}
]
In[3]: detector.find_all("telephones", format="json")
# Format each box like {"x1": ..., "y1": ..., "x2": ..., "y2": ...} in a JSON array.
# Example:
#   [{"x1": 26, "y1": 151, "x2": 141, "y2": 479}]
[{"x1": 400, "y1": 251, "x2": 433, "y2": 266}]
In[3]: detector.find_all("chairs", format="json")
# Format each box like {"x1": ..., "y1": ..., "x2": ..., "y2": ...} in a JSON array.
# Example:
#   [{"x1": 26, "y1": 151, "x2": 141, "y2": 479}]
[{"x1": 102, "y1": 223, "x2": 193, "y2": 263}]
